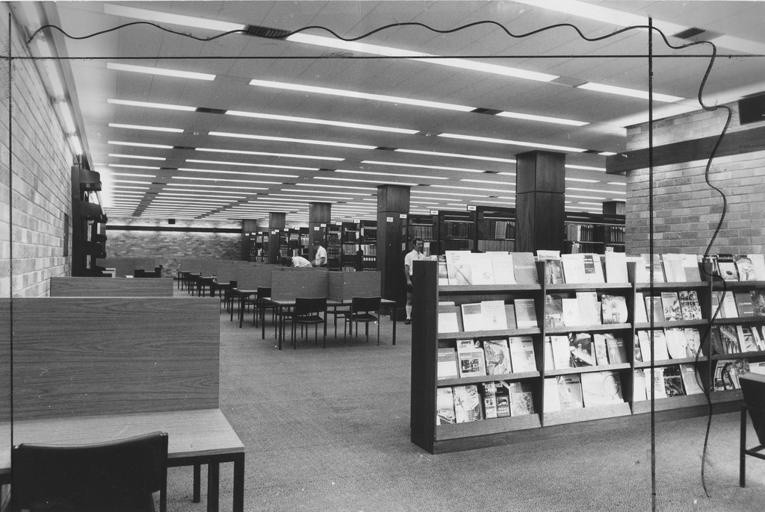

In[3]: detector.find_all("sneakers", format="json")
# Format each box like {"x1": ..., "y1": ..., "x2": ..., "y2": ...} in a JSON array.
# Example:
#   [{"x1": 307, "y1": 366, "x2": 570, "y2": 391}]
[{"x1": 404, "y1": 318, "x2": 411, "y2": 325}]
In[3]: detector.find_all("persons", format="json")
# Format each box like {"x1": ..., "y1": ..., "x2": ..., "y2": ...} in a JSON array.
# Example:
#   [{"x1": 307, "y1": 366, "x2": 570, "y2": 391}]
[
  {"x1": 283, "y1": 256, "x2": 313, "y2": 270},
  {"x1": 405, "y1": 238, "x2": 443, "y2": 324},
  {"x1": 311, "y1": 240, "x2": 328, "y2": 268}
]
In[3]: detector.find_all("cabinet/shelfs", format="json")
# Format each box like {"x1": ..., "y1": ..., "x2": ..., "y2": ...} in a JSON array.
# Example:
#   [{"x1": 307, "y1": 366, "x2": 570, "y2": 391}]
[
  {"x1": 71, "y1": 167, "x2": 102, "y2": 277},
  {"x1": 410, "y1": 260, "x2": 765, "y2": 454}
]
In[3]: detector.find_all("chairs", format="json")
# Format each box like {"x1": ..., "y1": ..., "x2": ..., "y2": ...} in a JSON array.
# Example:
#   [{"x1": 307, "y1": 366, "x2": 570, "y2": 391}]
[
  {"x1": 178, "y1": 257, "x2": 382, "y2": 348},
  {"x1": 10, "y1": 431, "x2": 167, "y2": 512},
  {"x1": 739, "y1": 373, "x2": 764, "y2": 487}
]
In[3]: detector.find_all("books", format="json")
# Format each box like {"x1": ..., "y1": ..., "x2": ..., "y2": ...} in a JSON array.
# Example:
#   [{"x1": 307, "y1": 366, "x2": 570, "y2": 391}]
[
  {"x1": 566, "y1": 223, "x2": 625, "y2": 253},
  {"x1": 435, "y1": 248, "x2": 765, "y2": 430},
  {"x1": 410, "y1": 221, "x2": 516, "y2": 255}
]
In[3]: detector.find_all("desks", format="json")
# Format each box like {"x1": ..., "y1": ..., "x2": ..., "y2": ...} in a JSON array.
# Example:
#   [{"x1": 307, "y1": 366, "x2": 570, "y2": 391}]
[{"x1": 11, "y1": 297, "x2": 245, "y2": 512}]
